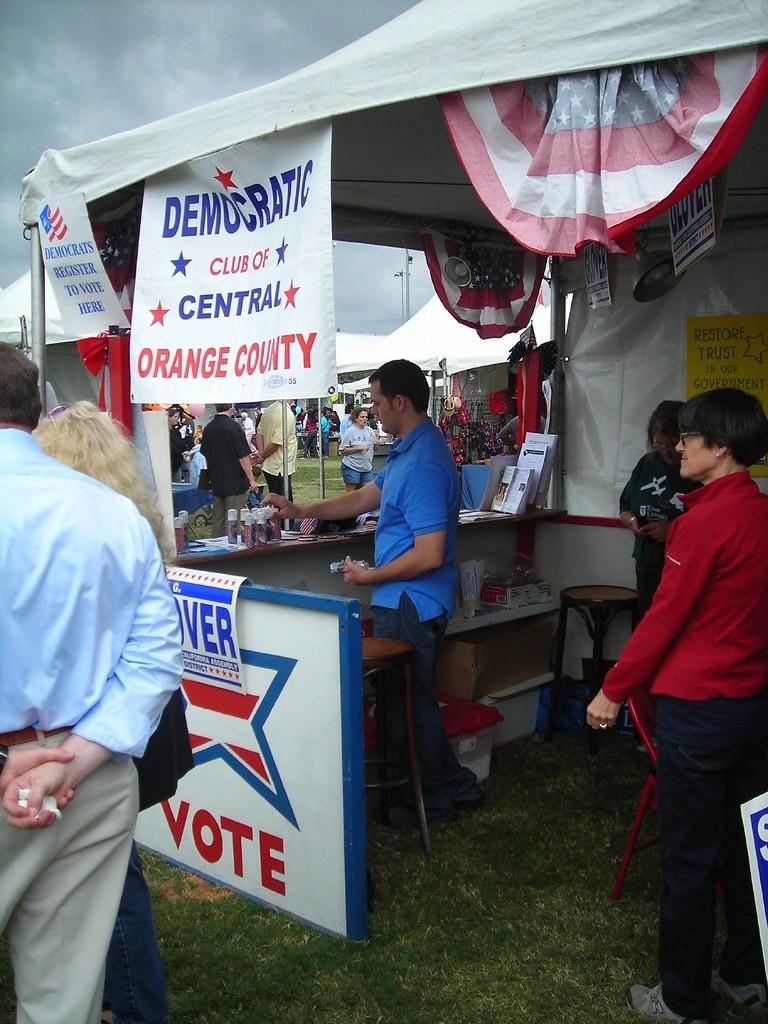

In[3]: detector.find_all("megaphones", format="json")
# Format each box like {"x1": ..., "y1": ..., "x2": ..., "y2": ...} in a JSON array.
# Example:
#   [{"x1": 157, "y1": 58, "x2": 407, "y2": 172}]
[{"x1": 444, "y1": 256, "x2": 472, "y2": 288}]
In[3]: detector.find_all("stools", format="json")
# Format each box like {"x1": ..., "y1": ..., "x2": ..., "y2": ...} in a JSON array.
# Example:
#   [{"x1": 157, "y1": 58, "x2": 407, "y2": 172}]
[
  {"x1": 546, "y1": 585, "x2": 642, "y2": 741},
  {"x1": 362, "y1": 639, "x2": 432, "y2": 853}
]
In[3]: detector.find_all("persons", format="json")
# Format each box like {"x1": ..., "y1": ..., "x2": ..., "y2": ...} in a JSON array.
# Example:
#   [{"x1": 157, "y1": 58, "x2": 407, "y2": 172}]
[
  {"x1": 260, "y1": 359, "x2": 485, "y2": 828},
  {"x1": 498, "y1": 415, "x2": 547, "y2": 448},
  {"x1": 0, "y1": 341, "x2": 185, "y2": 1024},
  {"x1": 617, "y1": 399, "x2": 701, "y2": 754},
  {"x1": 167, "y1": 400, "x2": 378, "y2": 538},
  {"x1": 587, "y1": 388, "x2": 768, "y2": 1024}
]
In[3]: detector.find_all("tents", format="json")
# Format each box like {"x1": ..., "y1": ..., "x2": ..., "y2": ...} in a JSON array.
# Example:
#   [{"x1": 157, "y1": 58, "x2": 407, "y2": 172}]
[
  {"x1": 0, "y1": 265, "x2": 289, "y2": 530},
  {"x1": 318, "y1": 255, "x2": 573, "y2": 507},
  {"x1": 19, "y1": 0, "x2": 768, "y2": 517}
]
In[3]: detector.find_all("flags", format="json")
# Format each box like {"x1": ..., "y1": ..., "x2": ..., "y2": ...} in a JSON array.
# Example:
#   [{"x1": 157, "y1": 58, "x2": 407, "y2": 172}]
[{"x1": 520, "y1": 320, "x2": 537, "y2": 349}]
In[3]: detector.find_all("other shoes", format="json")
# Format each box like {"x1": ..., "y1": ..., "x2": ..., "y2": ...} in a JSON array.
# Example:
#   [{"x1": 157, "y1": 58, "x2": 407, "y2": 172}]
[{"x1": 375, "y1": 805, "x2": 456, "y2": 827}]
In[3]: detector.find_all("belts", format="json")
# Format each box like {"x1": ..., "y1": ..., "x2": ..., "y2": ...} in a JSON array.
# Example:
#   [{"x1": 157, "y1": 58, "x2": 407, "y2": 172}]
[{"x1": 0, "y1": 722, "x2": 78, "y2": 745}]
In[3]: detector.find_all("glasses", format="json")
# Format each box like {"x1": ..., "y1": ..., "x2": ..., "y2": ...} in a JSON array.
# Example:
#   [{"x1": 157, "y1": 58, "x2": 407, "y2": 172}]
[
  {"x1": 651, "y1": 437, "x2": 676, "y2": 451},
  {"x1": 678, "y1": 429, "x2": 703, "y2": 444}
]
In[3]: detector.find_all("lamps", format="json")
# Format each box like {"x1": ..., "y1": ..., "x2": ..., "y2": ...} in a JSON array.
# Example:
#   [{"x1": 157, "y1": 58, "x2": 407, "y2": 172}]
[
  {"x1": 442, "y1": 232, "x2": 479, "y2": 286},
  {"x1": 628, "y1": 232, "x2": 686, "y2": 302}
]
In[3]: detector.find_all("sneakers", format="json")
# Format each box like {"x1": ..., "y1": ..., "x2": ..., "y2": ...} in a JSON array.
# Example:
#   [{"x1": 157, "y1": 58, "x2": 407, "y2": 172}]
[
  {"x1": 709, "y1": 966, "x2": 768, "y2": 1012},
  {"x1": 625, "y1": 982, "x2": 708, "y2": 1024}
]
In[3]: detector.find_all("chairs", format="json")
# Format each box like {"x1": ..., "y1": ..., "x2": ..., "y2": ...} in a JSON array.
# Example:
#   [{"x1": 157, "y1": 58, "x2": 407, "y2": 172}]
[{"x1": 610, "y1": 691, "x2": 728, "y2": 903}]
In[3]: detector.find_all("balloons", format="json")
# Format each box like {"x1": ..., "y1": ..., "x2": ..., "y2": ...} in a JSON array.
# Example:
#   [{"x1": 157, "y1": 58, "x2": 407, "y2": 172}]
[{"x1": 189, "y1": 403, "x2": 205, "y2": 417}]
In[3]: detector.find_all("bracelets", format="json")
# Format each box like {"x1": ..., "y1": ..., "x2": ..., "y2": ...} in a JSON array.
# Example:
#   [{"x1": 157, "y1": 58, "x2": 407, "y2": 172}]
[
  {"x1": 0, "y1": 743, "x2": 9, "y2": 775},
  {"x1": 627, "y1": 515, "x2": 636, "y2": 528}
]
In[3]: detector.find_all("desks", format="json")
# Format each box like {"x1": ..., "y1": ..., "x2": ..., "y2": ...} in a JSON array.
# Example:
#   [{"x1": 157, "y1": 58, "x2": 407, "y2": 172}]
[
  {"x1": 171, "y1": 483, "x2": 213, "y2": 540},
  {"x1": 462, "y1": 464, "x2": 491, "y2": 510}
]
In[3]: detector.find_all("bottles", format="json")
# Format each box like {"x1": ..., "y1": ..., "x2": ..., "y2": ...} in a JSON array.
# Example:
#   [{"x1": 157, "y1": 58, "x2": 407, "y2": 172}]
[
  {"x1": 330, "y1": 561, "x2": 375, "y2": 571},
  {"x1": 227, "y1": 509, "x2": 237, "y2": 544},
  {"x1": 240, "y1": 503, "x2": 281, "y2": 549},
  {"x1": 174, "y1": 517, "x2": 184, "y2": 553},
  {"x1": 179, "y1": 510, "x2": 189, "y2": 550}
]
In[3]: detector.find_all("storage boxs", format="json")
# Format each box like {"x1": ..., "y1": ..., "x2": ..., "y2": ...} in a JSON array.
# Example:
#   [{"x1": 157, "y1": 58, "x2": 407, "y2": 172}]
[
  {"x1": 329, "y1": 441, "x2": 340, "y2": 457},
  {"x1": 362, "y1": 628, "x2": 560, "y2": 813},
  {"x1": 480, "y1": 579, "x2": 553, "y2": 609}
]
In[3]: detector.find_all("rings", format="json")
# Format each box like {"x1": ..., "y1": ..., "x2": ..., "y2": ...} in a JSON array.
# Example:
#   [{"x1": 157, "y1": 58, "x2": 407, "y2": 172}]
[{"x1": 599, "y1": 723, "x2": 608, "y2": 729}]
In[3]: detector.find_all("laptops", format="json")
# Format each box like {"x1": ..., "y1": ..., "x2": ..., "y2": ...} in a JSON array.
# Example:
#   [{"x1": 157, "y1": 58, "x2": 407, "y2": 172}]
[{"x1": 197, "y1": 469, "x2": 212, "y2": 490}]
